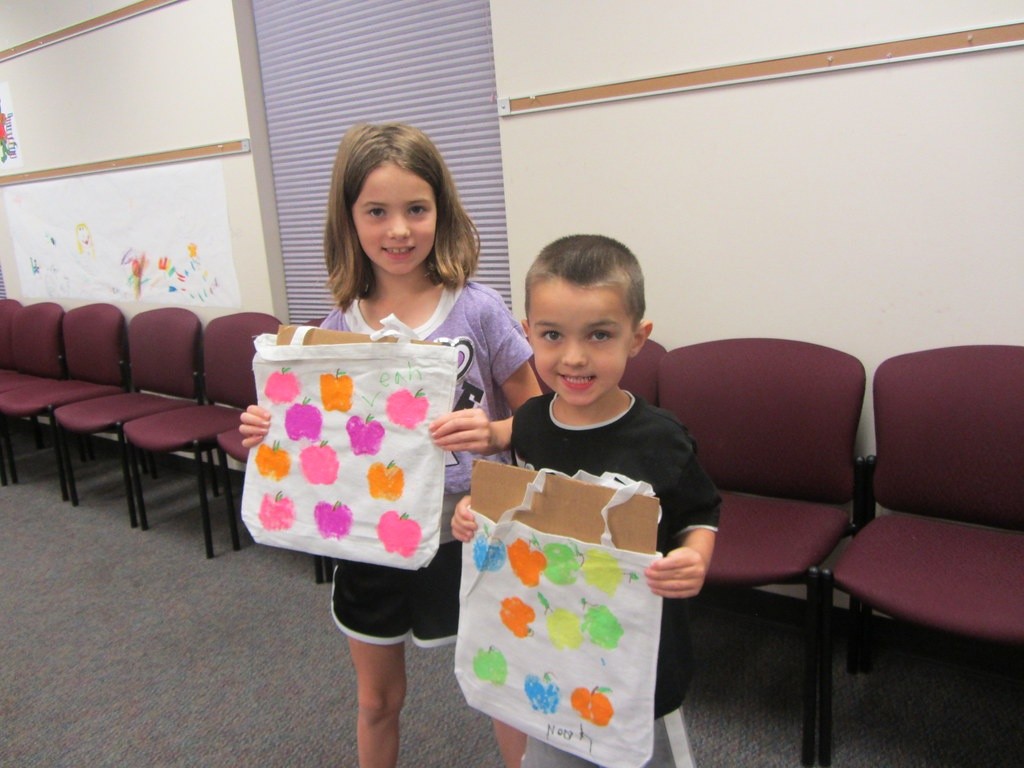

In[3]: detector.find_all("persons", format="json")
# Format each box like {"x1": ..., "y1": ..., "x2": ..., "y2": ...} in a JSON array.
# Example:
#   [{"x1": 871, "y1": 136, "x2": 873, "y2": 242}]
[
  {"x1": 449, "y1": 232, "x2": 724, "y2": 768},
  {"x1": 235, "y1": 121, "x2": 547, "y2": 768}
]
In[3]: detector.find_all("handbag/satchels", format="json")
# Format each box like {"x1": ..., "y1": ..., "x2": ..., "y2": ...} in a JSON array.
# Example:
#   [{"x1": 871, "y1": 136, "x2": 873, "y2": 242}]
[
  {"x1": 453, "y1": 472, "x2": 663, "y2": 768},
  {"x1": 241, "y1": 332, "x2": 462, "y2": 572}
]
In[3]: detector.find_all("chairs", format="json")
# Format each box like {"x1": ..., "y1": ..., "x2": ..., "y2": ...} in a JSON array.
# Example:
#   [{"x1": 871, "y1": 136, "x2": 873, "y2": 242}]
[{"x1": 0, "y1": 296, "x2": 1023, "y2": 766}]
